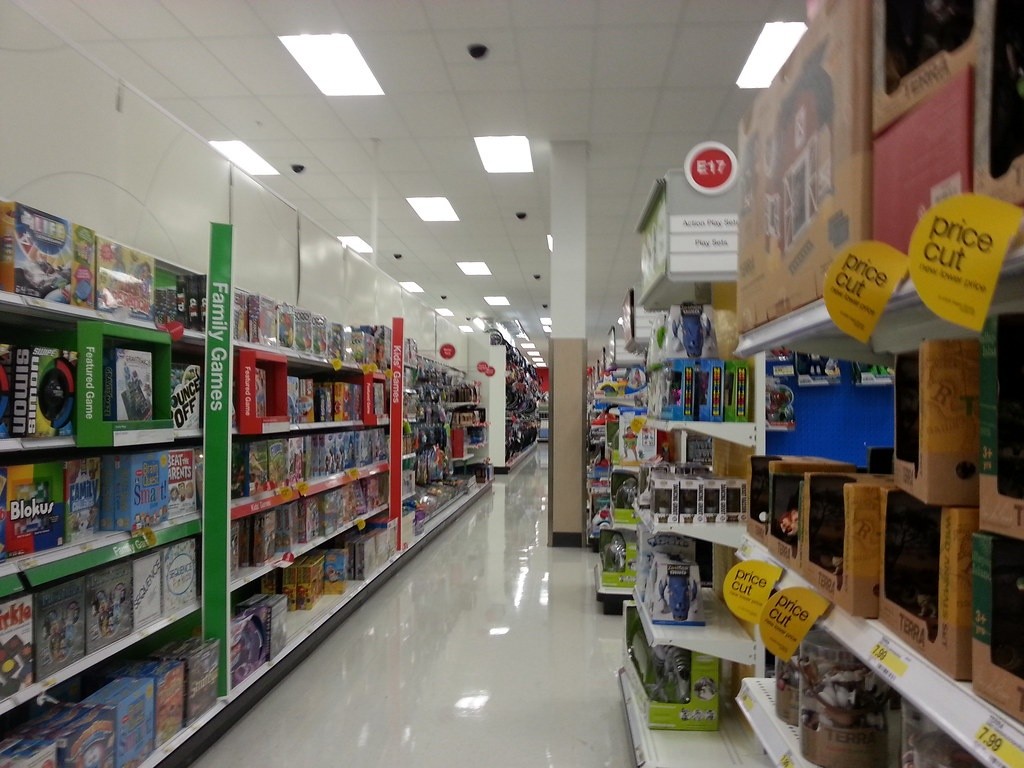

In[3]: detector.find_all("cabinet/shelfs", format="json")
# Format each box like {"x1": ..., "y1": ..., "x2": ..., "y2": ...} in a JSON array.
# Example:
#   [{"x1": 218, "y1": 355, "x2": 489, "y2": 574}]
[
  {"x1": 618, "y1": 252, "x2": 776, "y2": 768},
  {"x1": 734, "y1": 240, "x2": 1024, "y2": 768},
  {"x1": 0, "y1": 221, "x2": 417, "y2": 768},
  {"x1": 448, "y1": 402, "x2": 489, "y2": 460},
  {"x1": 488, "y1": 345, "x2": 549, "y2": 474},
  {"x1": 586, "y1": 360, "x2": 713, "y2": 615}
]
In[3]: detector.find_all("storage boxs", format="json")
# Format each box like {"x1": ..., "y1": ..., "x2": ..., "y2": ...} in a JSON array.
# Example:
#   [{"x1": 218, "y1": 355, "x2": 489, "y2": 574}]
[
  {"x1": 600, "y1": 527, "x2": 638, "y2": 587},
  {"x1": 231, "y1": 506, "x2": 416, "y2": 688},
  {"x1": 0, "y1": 342, "x2": 78, "y2": 439},
  {"x1": 231, "y1": 428, "x2": 414, "y2": 500},
  {"x1": 102, "y1": 348, "x2": 152, "y2": 422},
  {"x1": 0, "y1": 203, "x2": 154, "y2": 320},
  {"x1": 230, "y1": 474, "x2": 378, "y2": 581},
  {"x1": 747, "y1": 338, "x2": 1024, "y2": 722},
  {"x1": 613, "y1": 414, "x2": 657, "y2": 466},
  {"x1": 611, "y1": 466, "x2": 641, "y2": 525},
  {"x1": 171, "y1": 362, "x2": 199, "y2": 429},
  {"x1": 0, "y1": 638, "x2": 220, "y2": 768},
  {"x1": 623, "y1": 600, "x2": 719, "y2": 730},
  {"x1": 637, "y1": 525, "x2": 695, "y2": 601},
  {"x1": 639, "y1": 460, "x2": 747, "y2": 524},
  {"x1": 154, "y1": 274, "x2": 391, "y2": 369},
  {"x1": 232, "y1": 369, "x2": 384, "y2": 428},
  {"x1": 738, "y1": 0, "x2": 1024, "y2": 334},
  {"x1": 647, "y1": 359, "x2": 750, "y2": 422},
  {"x1": 0, "y1": 446, "x2": 203, "y2": 562},
  {"x1": 0, "y1": 538, "x2": 196, "y2": 701}
]
